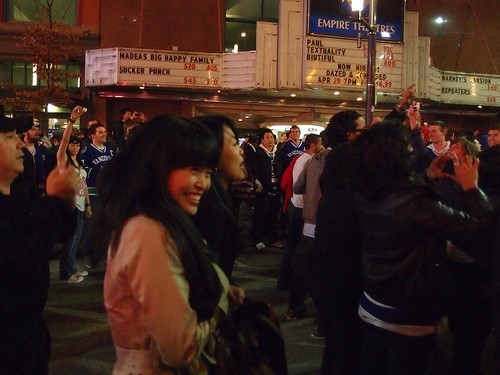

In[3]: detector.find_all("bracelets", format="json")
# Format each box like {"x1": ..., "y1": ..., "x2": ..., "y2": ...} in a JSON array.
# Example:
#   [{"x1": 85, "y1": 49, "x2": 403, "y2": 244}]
[
  {"x1": 70, "y1": 119, "x2": 75, "y2": 123},
  {"x1": 86, "y1": 203, "x2": 91, "y2": 206},
  {"x1": 398, "y1": 105, "x2": 405, "y2": 111}
]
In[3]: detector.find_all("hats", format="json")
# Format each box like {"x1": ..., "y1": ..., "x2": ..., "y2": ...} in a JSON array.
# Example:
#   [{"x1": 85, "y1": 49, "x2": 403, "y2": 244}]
[{"x1": 0, "y1": 114, "x2": 34, "y2": 134}]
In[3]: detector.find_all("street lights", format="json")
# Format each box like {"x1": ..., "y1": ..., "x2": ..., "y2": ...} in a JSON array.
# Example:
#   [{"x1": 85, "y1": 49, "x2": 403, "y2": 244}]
[
  {"x1": 347, "y1": 0, "x2": 377, "y2": 126},
  {"x1": 435, "y1": 16, "x2": 445, "y2": 70}
]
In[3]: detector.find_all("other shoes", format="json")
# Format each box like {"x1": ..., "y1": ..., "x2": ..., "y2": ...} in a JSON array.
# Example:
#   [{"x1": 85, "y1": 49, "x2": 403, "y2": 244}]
[
  {"x1": 287, "y1": 304, "x2": 308, "y2": 320},
  {"x1": 256, "y1": 242, "x2": 267, "y2": 252},
  {"x1": 311, "y1": 326, "x2": 326, "y2": 339},
  {"x1": 271, "y1": 241, "x2": 286, "y2": 248},
  {"x1": 61, "y1": 270, "x2": 88, "y2": 284}
]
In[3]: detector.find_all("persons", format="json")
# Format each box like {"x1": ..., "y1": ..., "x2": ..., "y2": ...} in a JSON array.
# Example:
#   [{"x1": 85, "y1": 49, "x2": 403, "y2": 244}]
[
  {"x1": 73, "y1": 114, "x2": 244, "y2": 374},
  {"x1": 197, "y1": 83, "x2": 500, "y2": 375},
  {"x1": 0, "y1": 106, "x2": 148, "y2": 375}
]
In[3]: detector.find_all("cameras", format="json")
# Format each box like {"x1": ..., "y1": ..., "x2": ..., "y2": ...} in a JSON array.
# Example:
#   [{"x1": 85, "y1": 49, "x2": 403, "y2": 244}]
[{"x1": 79, "y1": 107, "x2": 87, "y2": 113}]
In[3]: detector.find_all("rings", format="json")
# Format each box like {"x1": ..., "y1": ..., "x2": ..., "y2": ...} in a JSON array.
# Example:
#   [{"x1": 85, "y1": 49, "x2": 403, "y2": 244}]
[{"x1": 453, "y1": 162, "x2": 459, "y2": 166}]
